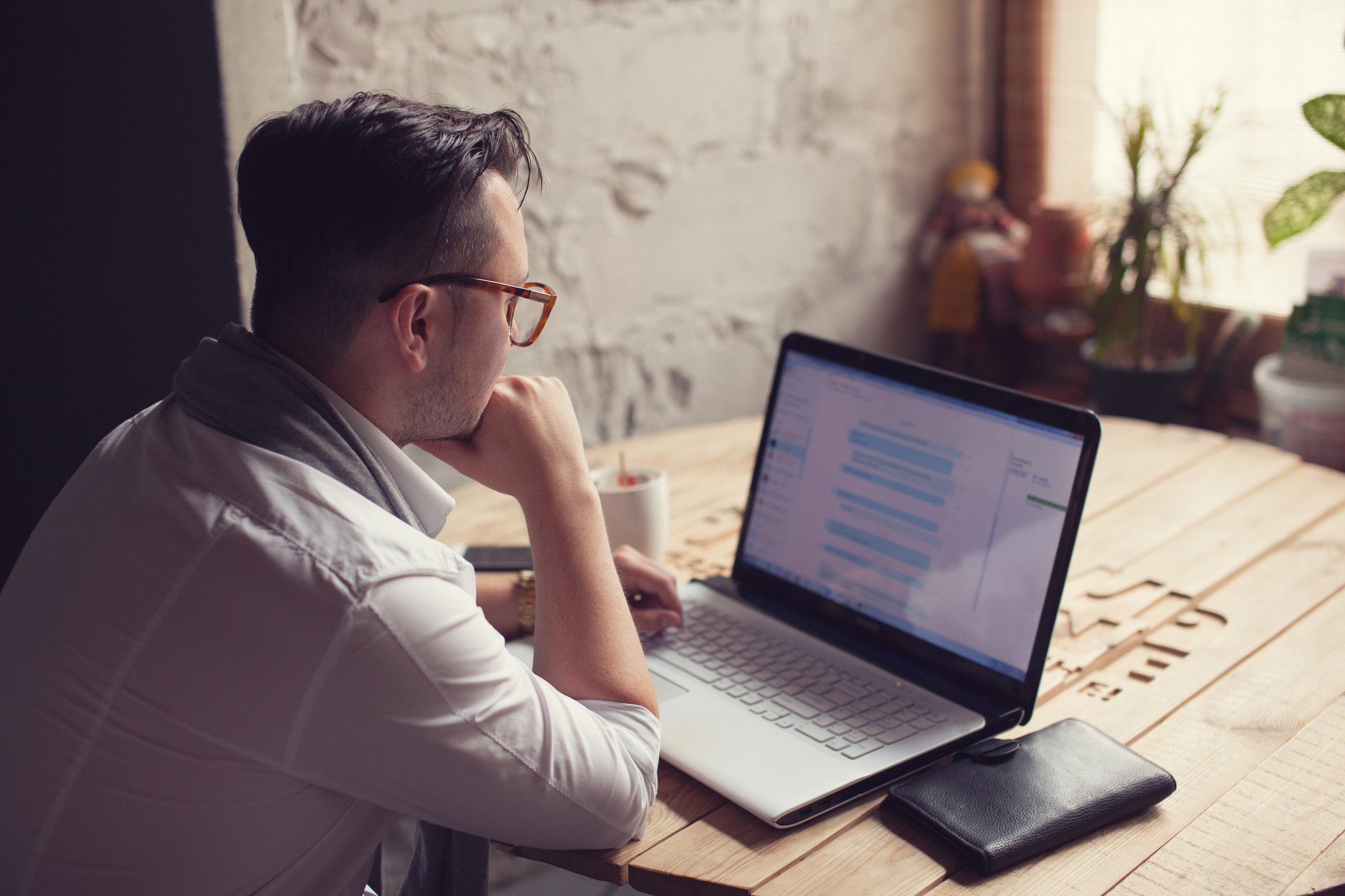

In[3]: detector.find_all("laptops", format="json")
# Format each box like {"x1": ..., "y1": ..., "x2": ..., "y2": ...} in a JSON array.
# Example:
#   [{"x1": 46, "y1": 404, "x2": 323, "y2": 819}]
[{"x1": 504, "y1": 329, "x2": 1103, "y2": 831}]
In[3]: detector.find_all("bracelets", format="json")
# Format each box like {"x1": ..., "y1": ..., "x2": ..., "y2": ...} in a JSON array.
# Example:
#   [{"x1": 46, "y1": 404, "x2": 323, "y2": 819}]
[{"x1": 513, "y1": 566, "x2": 538, "y2": 632}]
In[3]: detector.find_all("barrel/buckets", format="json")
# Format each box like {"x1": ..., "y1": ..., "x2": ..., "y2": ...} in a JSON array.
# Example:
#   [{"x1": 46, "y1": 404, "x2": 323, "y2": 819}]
[{"x1": 1250, "y1": 348, "x2": 1345, "y2": 473}]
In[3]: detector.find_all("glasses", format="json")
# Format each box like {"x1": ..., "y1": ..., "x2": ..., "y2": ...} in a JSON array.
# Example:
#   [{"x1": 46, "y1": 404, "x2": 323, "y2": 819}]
[{"x1": 374, "y1": 272, "x2": 555, "y2": 346}]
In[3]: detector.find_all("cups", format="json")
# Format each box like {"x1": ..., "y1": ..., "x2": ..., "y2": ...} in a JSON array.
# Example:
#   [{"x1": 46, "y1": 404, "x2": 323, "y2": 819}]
[{"x1": 591, "y1": 460, "x2": 667, "y2": 567}]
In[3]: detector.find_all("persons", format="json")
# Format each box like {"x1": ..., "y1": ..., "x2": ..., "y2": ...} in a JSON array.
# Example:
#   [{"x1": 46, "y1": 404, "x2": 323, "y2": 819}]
[
  {"x1": 4, "y1": 84, "x2": 684, "y2": 896},
  {"x1": 918, "y1": 157, "x2": 1032, "y2": 330}
]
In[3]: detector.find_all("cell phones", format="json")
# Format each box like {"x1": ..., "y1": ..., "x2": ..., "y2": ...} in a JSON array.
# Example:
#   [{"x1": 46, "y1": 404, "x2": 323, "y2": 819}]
[{"x1": 463, "y1": 545, "x2": 534, "y2": 570}]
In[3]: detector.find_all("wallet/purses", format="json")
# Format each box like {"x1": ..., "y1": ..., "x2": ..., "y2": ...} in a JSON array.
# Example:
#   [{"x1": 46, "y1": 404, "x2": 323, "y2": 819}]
[{"x1": 884, "y1": 717, "x2": 1177, "y2": 877}]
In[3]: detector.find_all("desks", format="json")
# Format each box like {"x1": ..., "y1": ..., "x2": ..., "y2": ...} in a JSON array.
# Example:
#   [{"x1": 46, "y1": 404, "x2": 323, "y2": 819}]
[{"x1": 428, "y1": 405, "x2": 1345, "y2": 896}]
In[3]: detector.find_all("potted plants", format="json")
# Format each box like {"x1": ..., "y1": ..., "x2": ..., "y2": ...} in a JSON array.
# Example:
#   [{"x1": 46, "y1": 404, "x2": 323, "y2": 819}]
[
  {"x1": 1067, "y1": 73, "x2": 1232, "y2": 415},
  {"x1": 1252, "y1": 292, "x2": 1345, "y2": 473}
]
